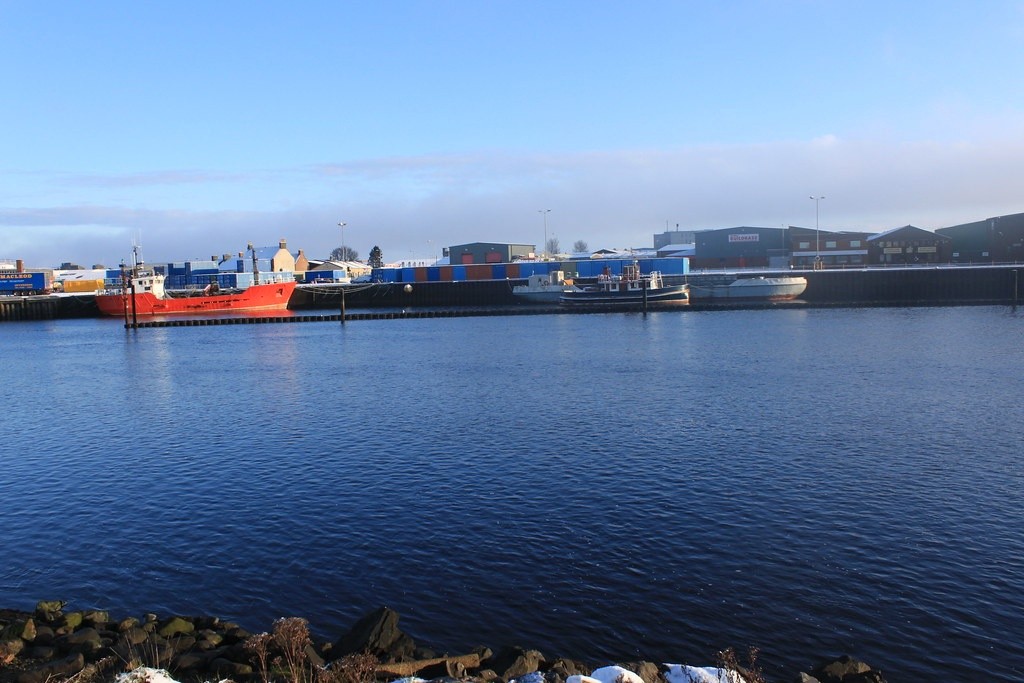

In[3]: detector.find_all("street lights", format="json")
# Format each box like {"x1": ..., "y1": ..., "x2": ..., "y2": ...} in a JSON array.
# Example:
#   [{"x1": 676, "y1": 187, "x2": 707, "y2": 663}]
[
  {"x1": 538, "y1": 209, "x2": 552, "y2": 260},
  {"x1": 338, "y1": 222, "x2": 347, "y2": 262},
  {"x1": 810, "y1": 195, "x2": 825, "y2": 262}
]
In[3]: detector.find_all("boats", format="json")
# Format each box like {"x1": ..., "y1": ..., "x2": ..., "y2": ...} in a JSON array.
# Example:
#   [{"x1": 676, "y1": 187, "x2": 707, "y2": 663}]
[
  {"x1": 93, "y1": 240, "x2": 298, "y2": 317},
  {"x1": 558, "y1": 260, "x2": 691, "y2": 310},
  {"x1": 512, "y1": 271, "x2": 807, "y2": 306}
]
